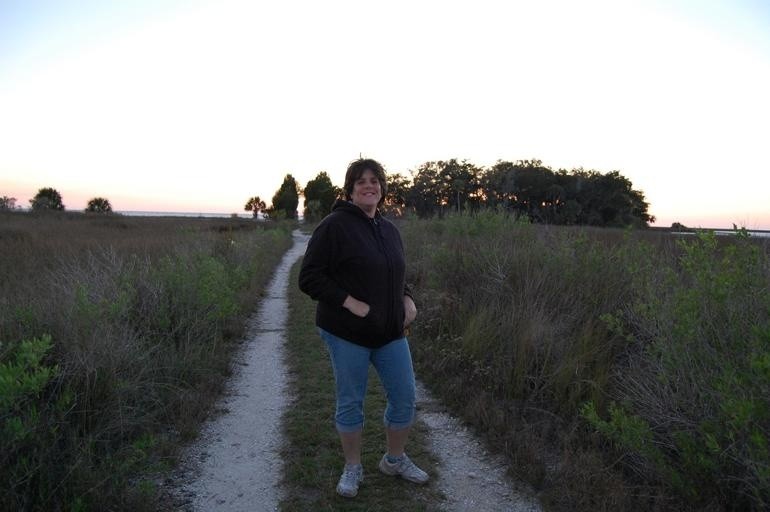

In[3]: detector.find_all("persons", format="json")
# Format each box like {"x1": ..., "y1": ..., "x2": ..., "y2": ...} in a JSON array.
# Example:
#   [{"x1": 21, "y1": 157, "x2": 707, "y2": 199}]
[{"x1": 298, "y1": 160, "x2": 428, "y2": 497}]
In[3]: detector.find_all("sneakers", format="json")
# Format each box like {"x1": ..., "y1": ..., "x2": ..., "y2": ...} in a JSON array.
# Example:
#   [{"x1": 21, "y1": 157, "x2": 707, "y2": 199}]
[
  {"x1": 379, "y1": 452, "x2": 429, "y2": 485},
  {"x1": 336, "y1": 463, "x2": 364, "y2": 498}
]
